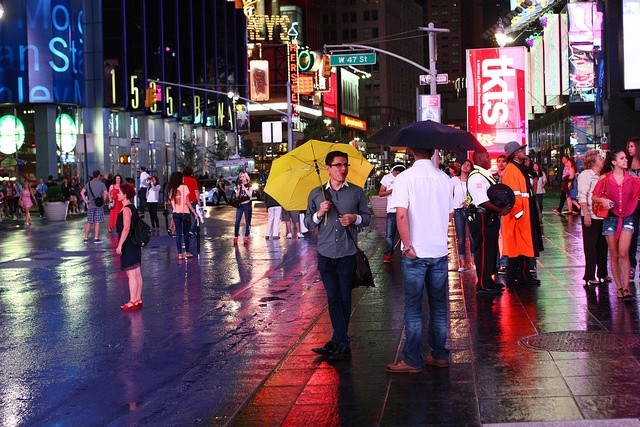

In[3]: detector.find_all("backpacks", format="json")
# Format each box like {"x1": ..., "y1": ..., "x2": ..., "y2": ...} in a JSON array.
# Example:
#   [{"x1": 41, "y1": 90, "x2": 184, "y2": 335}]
[{"x1": 130, "y1": 219, "x2": 151, "y2": 248}]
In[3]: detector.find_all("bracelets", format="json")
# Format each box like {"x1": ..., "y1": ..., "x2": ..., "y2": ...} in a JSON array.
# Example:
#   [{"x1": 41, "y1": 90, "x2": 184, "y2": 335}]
[{"x1": 583, "y1": 212, "x2": 590, "y2": 216}]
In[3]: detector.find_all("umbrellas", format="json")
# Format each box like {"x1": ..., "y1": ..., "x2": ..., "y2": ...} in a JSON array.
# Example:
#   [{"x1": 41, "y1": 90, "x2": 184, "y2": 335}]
[
  {"x1": 361, "y1": 119, "x2": 488, "y2": 155},
  {"x1": 262, "y1": 139, "x2": 375, "y2": 224},
  {"x1": 361, "y1": 124, "x2": 413, "y2": 169}
]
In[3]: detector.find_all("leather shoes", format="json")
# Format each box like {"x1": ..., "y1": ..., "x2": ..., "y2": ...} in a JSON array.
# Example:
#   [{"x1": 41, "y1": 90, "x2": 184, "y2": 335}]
[
  {"x1": 485, "y1": 281, "x2": 504, "y2": 289},
  {"x1": 519, "y1": 278, "x2": 540, "y2": 286},
  {"x1": 329, "y1": 343, "x2": 353, "y2": 361},
  {"x1": 586, "y1": 280, "x2": 601, "y2": 286},
  {"x1": 476, "y1": 286, "x2": 501, "y2": 296},
  {"x1": 311, "y1": 341, "x2": 329, "y2": 355}
]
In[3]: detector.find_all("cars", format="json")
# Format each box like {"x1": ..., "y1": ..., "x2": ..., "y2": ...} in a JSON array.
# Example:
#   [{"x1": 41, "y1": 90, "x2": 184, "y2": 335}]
[{"x1": 199, "y1": 178, "x2": 235, "y2": 206}]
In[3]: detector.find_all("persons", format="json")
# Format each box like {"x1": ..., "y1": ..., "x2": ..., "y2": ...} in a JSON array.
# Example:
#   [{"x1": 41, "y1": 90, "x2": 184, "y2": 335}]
[
  {"x1": 492, "y1": 154, "x2": 510, "y2": 275},
  {"x1": 142, "y1": 177, "x2": 161, "y2": 232},
  {"x1": 0, "y1": 192, "x2": 4, "y2": 223},
  {"x1": 499, "y1": 140, "x2": 544, "y2": 287},
  {"x1": 591, "y1": 150, "x2": 640, "y2": 298},
  {"x1": 61, "y1": 176, "x2": 71, "y2": 218},
  {"x1": 105, "y1": 173, "x2": 115, "y2": 214},
  {"x1": 303, "y1": 149, "x2": 371, "y2": 361},
  {"x1": 14, "y1": 180, "x2": 22, "y2": 216},
  {"x1": 234, "y1": 172, "x2": 253, "y2": 245},
  {"x1": 75, "y1": 176, "x2": 82, "y2": 213},
  {"x1": 195, "y1": 171, "x2": 233, "y2": 208},
  {"x1": 0, "y1": 180, "x2": 9, "y2": 217},
  {"x1": 84, "y1": 175, "x2": 91, "y2": 211},
  {"x1": 578, "y1": 150, "x2": 613, "y2": 284},
  {"x1": 138, "y1": 166, "x2": 152, "y2": 219},
  {"x1": 563, "y1": 160, "x2": 582, "y2": 215},
  {"x1": 531, "y1": 162, "x2": 548, "y2": 219},
  {"x1": 625, "y1": 138, "x2": 640, "y2": 280},
  {"x1": 115, "y1": 183, "x2": 144, "y2": 311},
  {"x1": 379, "y1": 161, "x2": 407, "y2": 263},
  {"x1": 36, "y1": 178, "x2": 48, "y2": 218},
  {"x1": 552, "y1": 155, "x2": 570, "y2": 213},
  {"x1": 524, "y1": 153, "x2": 543, "y2": 238},
  {"x1": 3, "y1": 182, "x2": 17, "y2": 218},
  {"x1": 170, "y1": 172, "x2": 198, "y2": 259},
  {"x1": 451, "y1": 158, "x2": 476, "y2": 272},
  {"x1": 264, "y1": 191, "x2": 282, "y2": 241},
  {"x1": 181, "y1": 166, "x2": 200, "y2": 236},
  {"x1": 18, "y1": 182, "x2": 38, "y2": 226},
  {"x1": 466, "y1": 147, "x2": 504, "y2": 297},
  {"x1": 282, "y1": 205, "x2": 305, "y2": 239},
  {"x1": 80, "y1": 170, "x2": 107, "y2": 244},
  {"x1": 385, "y1": 130, "x2": 455, "y2": 373},
  {"x1": 167, "y1": 172, "x2": 177, "y2": 237},
  {"x1": 46, "y1": 175, "x2": 57, "y2": 201},
  {"x1": 108, "y1": 174, "x2": 123, "y2": 233},
  {"x1": 99, "y1": 173, "x2": 103, "y2": 180},
  {"x1": 69, "y1": 177, "x2": 78, "y2": 214}
]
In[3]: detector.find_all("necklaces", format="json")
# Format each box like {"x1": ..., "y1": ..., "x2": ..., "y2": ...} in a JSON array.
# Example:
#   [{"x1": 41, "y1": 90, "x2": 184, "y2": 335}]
[{"x1": 115, "y1": 184, "x2": 120, "y2": 188}]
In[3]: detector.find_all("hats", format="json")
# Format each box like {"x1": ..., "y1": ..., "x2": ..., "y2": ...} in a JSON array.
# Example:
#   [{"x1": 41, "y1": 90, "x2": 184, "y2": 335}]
[
  {"x1": 487, "y1": 184, "x2": 515, "y2": 208},
  {"x1": 505, "y1": 141, "x2": 527, "y2": 160}
]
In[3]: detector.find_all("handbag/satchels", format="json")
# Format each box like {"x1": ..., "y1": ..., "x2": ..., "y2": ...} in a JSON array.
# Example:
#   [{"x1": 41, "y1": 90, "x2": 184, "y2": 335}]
[
  {"x1": 95, "y1": 196, "x2": 103, "y2": 207},
  {"x1": 229, "y1": 191, "x2": 241, "y2": 208},
  {"x1": 196, "y1": 204, "x2": 204, "y2": 224},
  {"x1": 352, "y1": 247, "x2": 375, "y2": 288},
  {"x1": 592, "y1": 189, "x2": 610, "y2": 219}
]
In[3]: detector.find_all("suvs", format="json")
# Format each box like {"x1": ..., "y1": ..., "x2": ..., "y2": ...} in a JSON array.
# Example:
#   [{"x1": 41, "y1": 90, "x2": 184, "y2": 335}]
[{"x1": 248, "y1": 173, "x2": 267, "y2": 200}]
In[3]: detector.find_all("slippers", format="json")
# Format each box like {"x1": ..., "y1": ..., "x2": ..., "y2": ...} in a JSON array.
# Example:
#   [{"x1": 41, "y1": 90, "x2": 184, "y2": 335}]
[
  {"x1": 622, "y1": 288, "x2": 633, "y2": 298},
  {"x1": 616, "y1": 288, "x2": 623, "y2": 298}
]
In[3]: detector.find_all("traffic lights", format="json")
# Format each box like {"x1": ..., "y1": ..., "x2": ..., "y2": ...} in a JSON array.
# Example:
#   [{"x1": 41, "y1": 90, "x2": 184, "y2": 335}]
[
  {"x1": 119, "y1": 156, "x2": 131, "y2": 164},
  {"x1": 323, "y1": 56, "x2": 332, "y2": 78},
  {"x1": 145, "y1": 88, "x2": 157, "y2": 107}
]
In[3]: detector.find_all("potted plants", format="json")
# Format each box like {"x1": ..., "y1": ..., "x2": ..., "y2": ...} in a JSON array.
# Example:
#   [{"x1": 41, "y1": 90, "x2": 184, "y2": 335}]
[{"x1": 42, "y1": 183, "x2": 70, "y2": 221}]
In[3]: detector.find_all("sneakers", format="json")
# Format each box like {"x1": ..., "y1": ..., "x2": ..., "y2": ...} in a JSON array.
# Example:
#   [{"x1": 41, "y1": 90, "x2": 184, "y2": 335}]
[
  {"x1": 186, "y1": 252, "x2": 194, "y2": 258},
  {"x1": 273, "y1": 236, "x2": 280, "y2": 238},
  {"x1": 286, "y1": 232, "x2": 292, "y2": 239},
  {"x1": 83, "y1": 239, "x2": 88, "y2": 244},
  {"x1": 297, "y1": 233, "x2": 304, "y2": 238},
  {"x1": 384, "y1": 255, "x2": 392, "y2": 262},
  {"x1": 178, "y1": 253, "x2": 185, "y2": 259},
  {"x1": 265, "y1": 236, "x2": 270, "y2": 239},
  {"x1": 94, "y1": 238, "x2": 104, "y2": 245},
  {"x1": 385, "y1": 361, "x2": 422, "y2": 373},
  {"x1": 425, "y1": 354, "x2": 449, "y2": 368}
]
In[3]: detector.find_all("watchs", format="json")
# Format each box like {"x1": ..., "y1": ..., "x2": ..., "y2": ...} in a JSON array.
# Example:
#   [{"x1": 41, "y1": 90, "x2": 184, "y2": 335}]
[{"x1": 403, "y1": 245, "x2": 412, "y2": 253}]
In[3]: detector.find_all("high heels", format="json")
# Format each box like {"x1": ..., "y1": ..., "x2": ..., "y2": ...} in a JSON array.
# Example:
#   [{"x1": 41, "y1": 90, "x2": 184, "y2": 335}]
[{"x1": 599, "y1": 276, "x2": 613, "y2": 282}]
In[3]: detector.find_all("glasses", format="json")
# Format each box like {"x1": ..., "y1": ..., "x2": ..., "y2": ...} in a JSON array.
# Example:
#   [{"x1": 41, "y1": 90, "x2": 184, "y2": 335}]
[{"x1": 331, "y1": 163, "x2": 351, "y2": 167}]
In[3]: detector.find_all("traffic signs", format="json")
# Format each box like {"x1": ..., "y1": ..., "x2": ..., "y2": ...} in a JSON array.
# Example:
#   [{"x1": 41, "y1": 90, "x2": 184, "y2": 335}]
[
  {"x1": 330, "y1": 53, "x2": 377, "y2": 65},
  {"x1": 420, "y1": 73, "x2": 447, "y2": 84},
  {"x1": 415, "y1": 94, "x2": 443, "y2": 125}
]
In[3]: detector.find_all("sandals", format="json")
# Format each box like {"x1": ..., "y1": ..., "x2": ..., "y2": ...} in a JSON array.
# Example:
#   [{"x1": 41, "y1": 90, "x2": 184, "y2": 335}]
[{"x1": 121, "y1": 299, "x2": 144, "y2": 312}]
